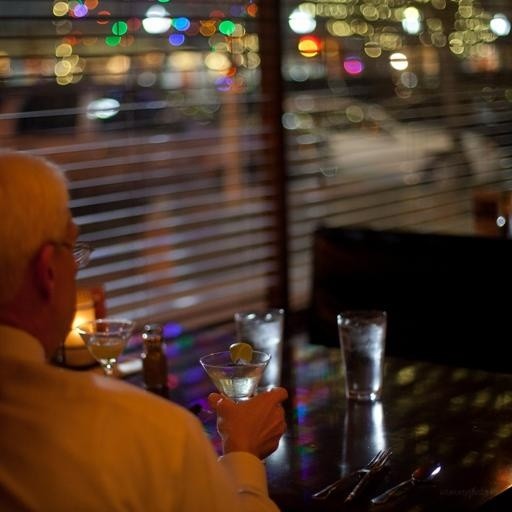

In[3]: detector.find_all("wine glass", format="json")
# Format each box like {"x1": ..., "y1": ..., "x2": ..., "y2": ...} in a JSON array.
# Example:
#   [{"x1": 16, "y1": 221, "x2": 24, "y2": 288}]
[{"x1": 77, "y1": 318, "x2": 138, "y2": 378}]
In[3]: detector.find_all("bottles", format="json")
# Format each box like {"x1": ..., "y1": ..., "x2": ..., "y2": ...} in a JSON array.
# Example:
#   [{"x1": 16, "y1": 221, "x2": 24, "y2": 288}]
[{"x1": 140, "y1": 323, "x2": 169, "y2": 397}]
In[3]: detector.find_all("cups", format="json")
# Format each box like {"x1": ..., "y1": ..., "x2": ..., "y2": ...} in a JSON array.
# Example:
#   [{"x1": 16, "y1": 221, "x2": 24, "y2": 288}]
[
  {"x1": 200, "y1": 350, "x2": 270, "y2": 401},
  {"x1": 337, "y1": 310, "x2": 388, "y2": 401},
  {"x1": 234, "y1": 310, "x2": 283, "y2": 390}
]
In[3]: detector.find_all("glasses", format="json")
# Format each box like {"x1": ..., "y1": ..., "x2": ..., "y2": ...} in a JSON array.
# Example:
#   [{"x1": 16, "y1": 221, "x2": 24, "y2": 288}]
[{"x1": 61, "y1": 241, "x2": 94, "y2": 269}]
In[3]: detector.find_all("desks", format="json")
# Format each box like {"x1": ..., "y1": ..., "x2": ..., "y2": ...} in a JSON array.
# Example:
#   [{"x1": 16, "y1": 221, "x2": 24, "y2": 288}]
[{"x1": 118, "y1": 323, "x2": 509, "y2": 512}]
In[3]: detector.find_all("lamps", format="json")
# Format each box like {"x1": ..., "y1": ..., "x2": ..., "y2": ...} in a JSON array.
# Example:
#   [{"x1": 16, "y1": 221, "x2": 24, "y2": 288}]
[{"x1": 65, "y1": 284, "x2": 107, "y2": 367}]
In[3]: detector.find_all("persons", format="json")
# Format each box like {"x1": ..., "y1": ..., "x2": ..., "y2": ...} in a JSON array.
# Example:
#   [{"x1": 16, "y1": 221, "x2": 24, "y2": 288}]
[{"x1": 0, "y1": 149, "x2": 292, "y2": 512}]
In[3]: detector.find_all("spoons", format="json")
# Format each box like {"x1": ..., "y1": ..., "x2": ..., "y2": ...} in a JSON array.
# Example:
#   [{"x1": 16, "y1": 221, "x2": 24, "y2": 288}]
[{"x1": 370, "y1": 463, "x2": 442, "y2": 502}]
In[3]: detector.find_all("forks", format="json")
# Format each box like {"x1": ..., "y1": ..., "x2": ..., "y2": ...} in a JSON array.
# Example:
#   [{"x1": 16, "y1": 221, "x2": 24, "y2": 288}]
[{"x1": 312, "y1": 449, "x2": 391, "y2": 503}]
